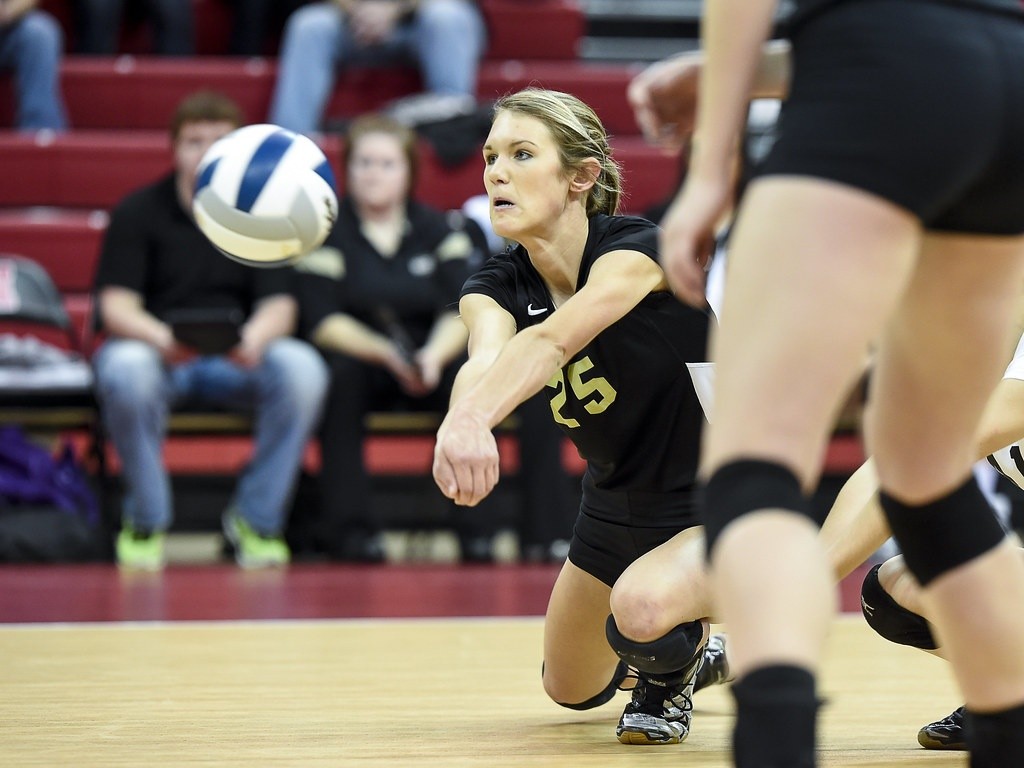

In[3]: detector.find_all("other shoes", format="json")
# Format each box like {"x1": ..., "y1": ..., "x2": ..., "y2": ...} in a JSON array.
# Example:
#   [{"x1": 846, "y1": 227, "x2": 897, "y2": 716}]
[
  {"x1": 115, "y1": 515, "x2": 169, "y2": 572},
  {"x1": 222, "y1": 506, "x2": 290, "y2": 570},
  {"x1": 917, "y1": 703, "x2": 970, "y2": 750}
]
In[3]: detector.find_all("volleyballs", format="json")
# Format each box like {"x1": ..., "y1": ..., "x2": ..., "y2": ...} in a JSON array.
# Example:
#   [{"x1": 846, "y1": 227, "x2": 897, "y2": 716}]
[{"x1": 191, "y1": 125, "x2": 339, "y2": 270}]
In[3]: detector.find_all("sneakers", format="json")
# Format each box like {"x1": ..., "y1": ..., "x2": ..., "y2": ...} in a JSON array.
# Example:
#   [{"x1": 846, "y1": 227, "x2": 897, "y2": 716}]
[
  {"x1": 704, "y1": 632, "x2": 735, "y2": 684},
  {"x1": 615, "y1": 637, "x2": 710, "y2": 745}
]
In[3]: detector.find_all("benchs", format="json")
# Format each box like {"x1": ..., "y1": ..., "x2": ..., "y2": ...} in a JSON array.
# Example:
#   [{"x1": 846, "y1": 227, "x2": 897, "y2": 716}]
[{"x1": 0, "y1": 1, "x2": 867, "y2": 478}]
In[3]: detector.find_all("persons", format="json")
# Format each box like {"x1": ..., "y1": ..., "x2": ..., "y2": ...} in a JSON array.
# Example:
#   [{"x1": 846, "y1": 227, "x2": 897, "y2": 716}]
[
  {"x1": 433, "y1": 88, "x2": 734, "y2": 744},
  {"x1": 0, "y1": 1, "x2": 1024, "y2": 767}
]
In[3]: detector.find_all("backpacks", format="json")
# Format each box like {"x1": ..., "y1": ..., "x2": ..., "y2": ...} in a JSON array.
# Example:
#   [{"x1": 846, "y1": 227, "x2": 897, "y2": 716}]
[{"x1": 0, "y1": 428, "x2": 109, "y2": 564}]
